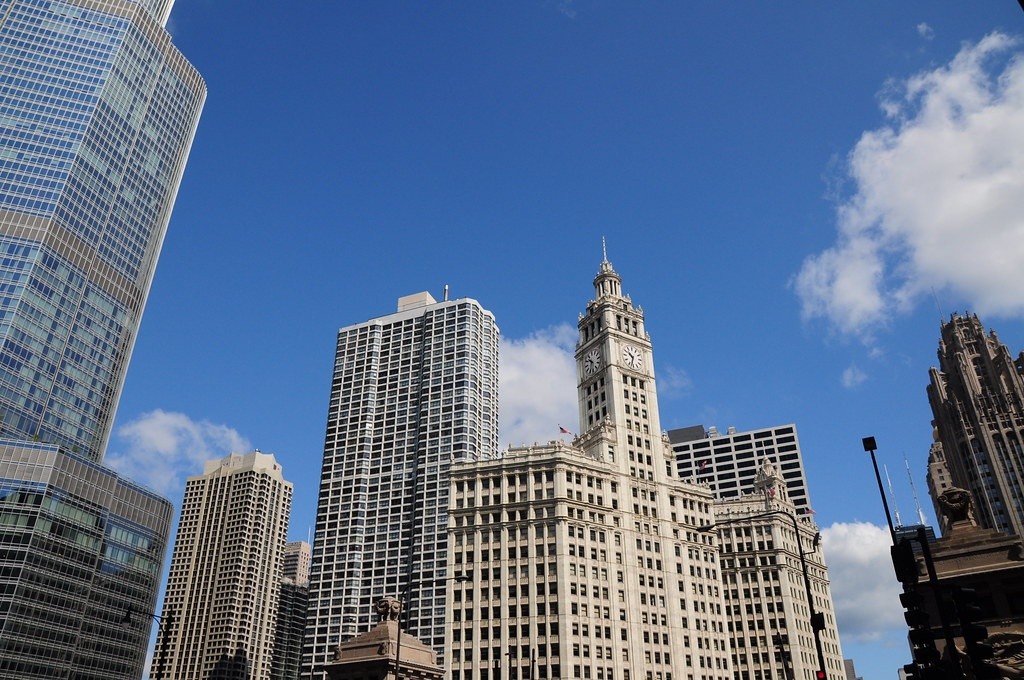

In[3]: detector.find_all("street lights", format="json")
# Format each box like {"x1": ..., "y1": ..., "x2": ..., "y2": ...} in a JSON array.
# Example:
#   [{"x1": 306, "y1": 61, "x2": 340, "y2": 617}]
[
  {"x1": 120, "y1": 604, "x2": 175, "y2": 680},
  {"x1": 697, "y1": 510, "x2": 827, "y2": 680},
  {"x1": 394, "y1": 574, "x2": 470, "y2": 680},
  {"x1": 863, "y1": 436, "x2": 930, "y2": 669}
]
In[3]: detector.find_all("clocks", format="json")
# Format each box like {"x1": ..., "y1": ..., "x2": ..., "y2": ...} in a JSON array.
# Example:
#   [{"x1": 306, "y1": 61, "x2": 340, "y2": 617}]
[
  {"x1": 623, "y1": 346, "x2": 643, "y2": 371},
  {"x1": 582, "y1": 350, "x2": 601, "y2": 376}
]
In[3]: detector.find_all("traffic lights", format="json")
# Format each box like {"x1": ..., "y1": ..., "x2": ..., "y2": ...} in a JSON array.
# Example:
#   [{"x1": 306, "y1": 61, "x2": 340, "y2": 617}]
[
  {"x1": 903, "y1": 662, "x2": 922, "y2": 680},
  {"x1": 815, "y1": 671, "x2": 827, "y2": 680}
]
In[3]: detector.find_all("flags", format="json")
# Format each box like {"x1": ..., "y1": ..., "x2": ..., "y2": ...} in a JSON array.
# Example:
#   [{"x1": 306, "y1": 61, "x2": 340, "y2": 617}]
[{"x1": 560, "y1": 426, "x2": 571, "y2": 433}]
